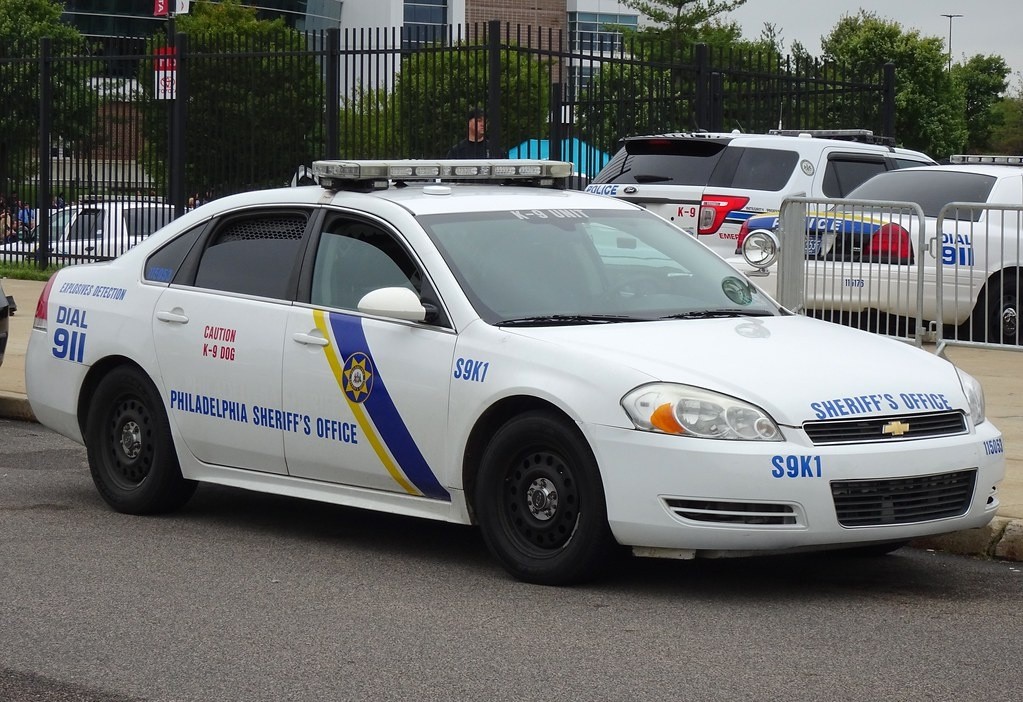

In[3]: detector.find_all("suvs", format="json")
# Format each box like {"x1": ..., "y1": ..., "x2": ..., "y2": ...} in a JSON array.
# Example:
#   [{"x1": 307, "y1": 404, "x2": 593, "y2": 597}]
[{"x1": 584, "y1": 129, "x2": 951, "y2": 311}]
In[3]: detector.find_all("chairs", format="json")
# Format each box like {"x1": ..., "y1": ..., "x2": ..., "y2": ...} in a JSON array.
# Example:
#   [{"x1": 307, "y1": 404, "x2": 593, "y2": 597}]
[{"x1": 338, "y1": 231, "x2": 433, "y2": 329}]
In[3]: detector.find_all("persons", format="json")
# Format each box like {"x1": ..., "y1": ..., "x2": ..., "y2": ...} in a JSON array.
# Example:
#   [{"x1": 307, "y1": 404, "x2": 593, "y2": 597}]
[
  {"x1": 444, "y1": 108, "x2": 507, "y2": 185},
  {"x1": 0, "y1": 187, "x2": 70, "y2": 246},
  {"x1": 134, "y1": 185, "x2": 216, "y2": 216}
]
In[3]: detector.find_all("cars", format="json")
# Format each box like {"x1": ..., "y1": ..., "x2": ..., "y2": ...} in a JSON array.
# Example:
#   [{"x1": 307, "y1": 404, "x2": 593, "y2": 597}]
[
  {"x1": 0, "y1": 195, "x2": 198, "y2": 264},
  {"x1": 0, "y1": 279, "x2": 18, "y2": 369},
  {"x1": 723, "y1": 152, "x2": 1023, "y2": 347},
  {"x1": 24, "y1": 156, "x2": 1005, "y2": 589}
]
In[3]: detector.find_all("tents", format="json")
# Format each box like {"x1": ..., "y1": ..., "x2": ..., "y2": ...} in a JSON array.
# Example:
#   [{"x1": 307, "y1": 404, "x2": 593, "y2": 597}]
[{"x1": 508, "y1": 137, "x2": 615, "y2": 179}]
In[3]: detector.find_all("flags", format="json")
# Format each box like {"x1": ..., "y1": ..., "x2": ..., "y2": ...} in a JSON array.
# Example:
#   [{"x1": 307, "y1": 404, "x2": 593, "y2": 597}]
[
  {"x1": 176, "y1": 0, "x2": 189, "y2": 14},
  {"x1": 153, "y1": 0, "x2": 169, "y2": 16}
]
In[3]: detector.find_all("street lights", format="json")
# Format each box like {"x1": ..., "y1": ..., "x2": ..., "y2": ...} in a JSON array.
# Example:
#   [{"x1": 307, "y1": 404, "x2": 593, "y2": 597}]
[{"x1": 940, "y1": 13, "x2": 964, "y2": 74}]
